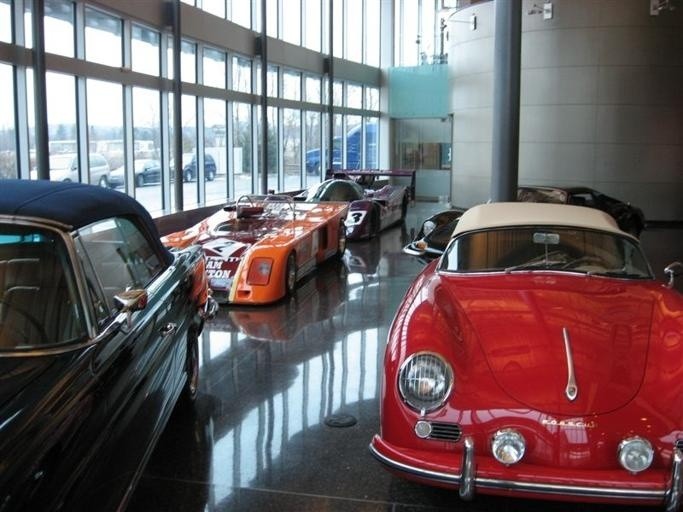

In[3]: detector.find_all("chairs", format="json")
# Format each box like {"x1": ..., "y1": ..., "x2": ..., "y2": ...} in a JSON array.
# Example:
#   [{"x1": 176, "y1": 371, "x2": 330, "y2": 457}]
[{"x1": 0, "y1": 258, "x2": 79, "y2": 345}]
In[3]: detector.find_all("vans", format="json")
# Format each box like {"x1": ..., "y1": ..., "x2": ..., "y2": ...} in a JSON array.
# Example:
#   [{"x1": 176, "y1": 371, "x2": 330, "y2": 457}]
[{"x1": 30, "y1": 152, "x2": 109, "y2": 188}]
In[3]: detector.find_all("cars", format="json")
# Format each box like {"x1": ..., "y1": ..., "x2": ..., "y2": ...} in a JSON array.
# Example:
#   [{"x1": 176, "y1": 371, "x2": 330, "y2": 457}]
[
  {"x1": 169, "y1": 152, "x2": 216, "y2": 182},
  {"x1": 403, "y1": 184, "x2": 646, "y2": 265},
  {"x1": 368, "y1": 201, "x2": 683, "y2": 511},
  {"x1": 0, "y1": 179, "x2": 218, "y2": 511},
  {"x1": 110, "y1": 158, "x2": 160, "y2": 186}
]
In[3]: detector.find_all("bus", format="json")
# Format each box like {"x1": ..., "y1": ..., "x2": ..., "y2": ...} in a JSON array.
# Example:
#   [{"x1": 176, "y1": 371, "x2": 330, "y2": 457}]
[{"x1": 306, "y1": 122, "x2": 377, "y2": 175}]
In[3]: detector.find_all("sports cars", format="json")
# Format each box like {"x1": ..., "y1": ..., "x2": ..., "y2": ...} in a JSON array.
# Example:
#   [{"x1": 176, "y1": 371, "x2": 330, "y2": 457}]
[
  {"x1": 274, "y1": 167, "x2": 417, "y2": 241},
  {"x1": 160, "y1": 190, "x2": 350, "y2": 306}
]
